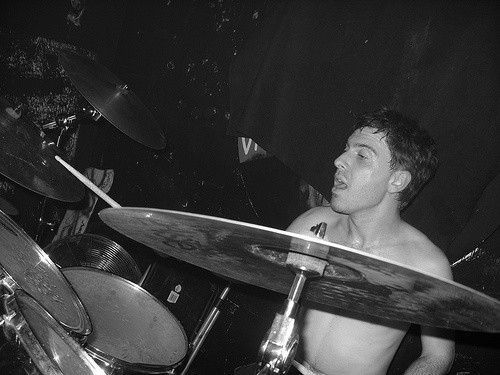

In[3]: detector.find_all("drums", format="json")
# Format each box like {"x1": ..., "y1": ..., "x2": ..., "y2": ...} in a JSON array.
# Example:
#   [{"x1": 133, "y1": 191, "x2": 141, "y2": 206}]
[
  {"x1": 0, "y1": 209, "x2": 93, "y2": 347},
  {"x1": 61, "y1": 267, "x2": 188, "y2": 375},
  {"x1": 0, "y1": 290, "x2": 101, "y2": 375}
]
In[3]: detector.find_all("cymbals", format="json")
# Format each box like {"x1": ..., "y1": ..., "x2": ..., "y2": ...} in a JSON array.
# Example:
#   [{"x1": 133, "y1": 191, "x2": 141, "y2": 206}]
[
  {"x1": 60, "y1": 54, "x2": 165, "y2": 148},
  {"x1": 0, "y1": 97, "x2": 84, "y2": 203},
  {"x1": 98, "y1": 207, "x2": 500, "y2": 335}
]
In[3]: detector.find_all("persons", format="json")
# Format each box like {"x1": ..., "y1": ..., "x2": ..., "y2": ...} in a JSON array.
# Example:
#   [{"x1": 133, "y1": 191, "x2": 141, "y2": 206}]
[
  {"x1": 233, "y1": 105, "x2": 455, "y2": 375},
  {"x1": 0, "y1": 0, "x2": 104, "y2": 164}
]
